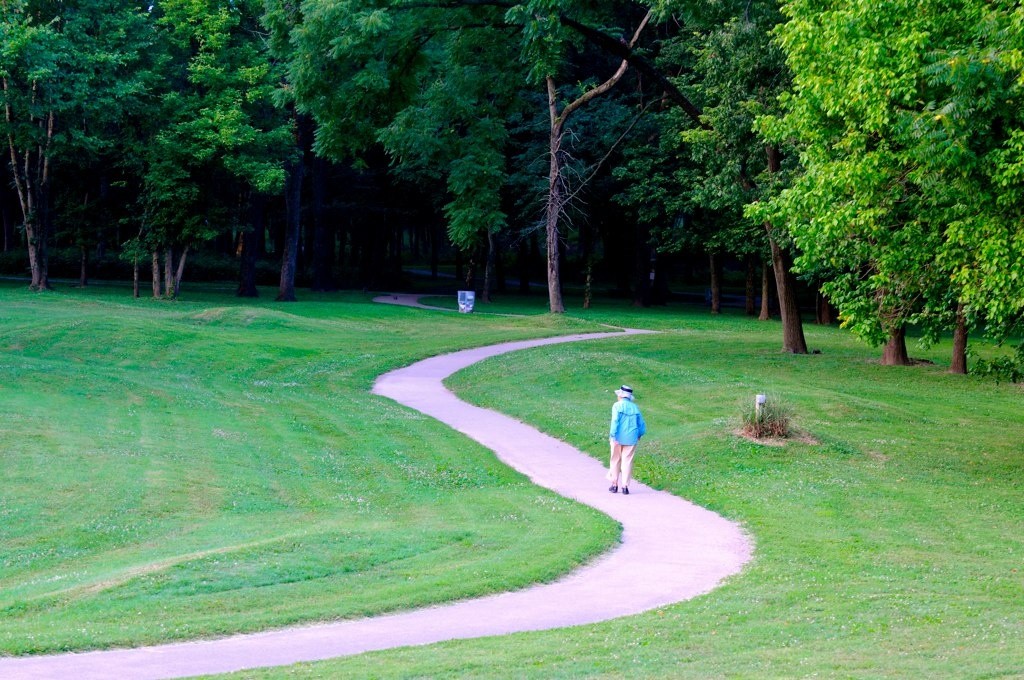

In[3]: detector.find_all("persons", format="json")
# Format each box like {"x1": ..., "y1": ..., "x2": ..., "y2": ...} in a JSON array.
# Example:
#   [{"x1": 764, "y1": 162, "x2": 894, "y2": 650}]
[{"x1": 609, "y1": 385, "x2": 646, "y2": 494}]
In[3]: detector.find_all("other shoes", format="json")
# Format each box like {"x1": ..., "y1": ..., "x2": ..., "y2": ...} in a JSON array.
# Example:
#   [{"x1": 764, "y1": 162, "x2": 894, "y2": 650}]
[
  {"x1": 609, "y1": 486, "x2": 618, "y2": 493},
  {"x1": 622, "y1": 488, "x2": 629, "y2": 494}
]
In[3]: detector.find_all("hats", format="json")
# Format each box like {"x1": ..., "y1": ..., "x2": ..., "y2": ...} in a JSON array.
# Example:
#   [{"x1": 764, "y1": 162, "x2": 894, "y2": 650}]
[{"x1": 614, "y1": 385, "x2": 633, "y2": 399}]
